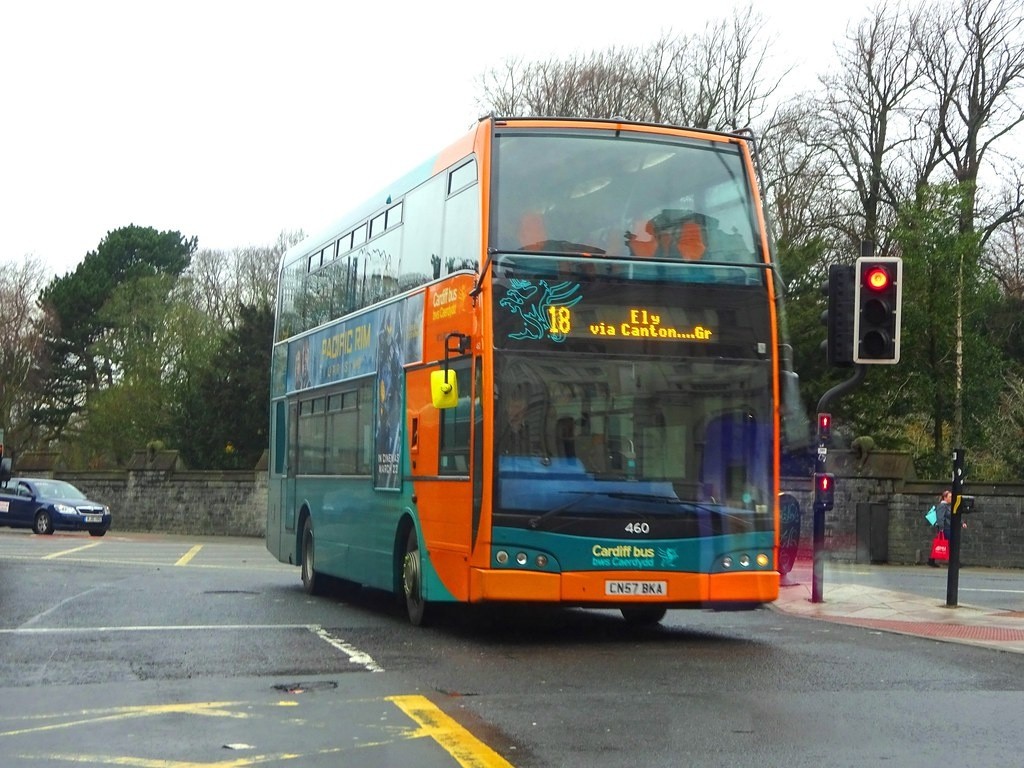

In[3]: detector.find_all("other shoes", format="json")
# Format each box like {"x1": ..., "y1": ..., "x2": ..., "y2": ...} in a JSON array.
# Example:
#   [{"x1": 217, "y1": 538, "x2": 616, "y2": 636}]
[{"x1": 928, "y1": 558, "x2": 940, "y2": 567}]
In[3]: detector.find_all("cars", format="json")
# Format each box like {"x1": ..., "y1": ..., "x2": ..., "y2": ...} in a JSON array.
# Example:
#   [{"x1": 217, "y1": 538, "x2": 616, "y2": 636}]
[{"x1": 0, "y1": 477, "x2": 112, "y2": 536}]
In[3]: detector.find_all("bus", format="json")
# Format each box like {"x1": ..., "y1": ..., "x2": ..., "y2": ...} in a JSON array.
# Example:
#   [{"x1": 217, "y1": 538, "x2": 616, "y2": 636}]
[{"x1": 263, "y1": 117, "x2": 801, "y2": 630}]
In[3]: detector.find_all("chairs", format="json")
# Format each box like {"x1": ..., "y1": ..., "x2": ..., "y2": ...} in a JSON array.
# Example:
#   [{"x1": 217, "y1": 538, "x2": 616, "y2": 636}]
[{"x1": 515, "y1": 208, "x2": 705, "y2": 260}]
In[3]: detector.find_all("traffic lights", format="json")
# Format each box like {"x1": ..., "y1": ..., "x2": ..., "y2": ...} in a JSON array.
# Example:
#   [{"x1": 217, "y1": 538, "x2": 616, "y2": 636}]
[
  {"x1": 814, "y1": 472, "x2": 835, "y2": 506},
  {"x1": 819, "y1": 264, "x2": 855, "y2": 368},
  {"x1": 853, "y1": 256, "x2": 902, "y2": 364},
  {"x1": 818, "y1": 413, "x2": 831, "y2": 436}
]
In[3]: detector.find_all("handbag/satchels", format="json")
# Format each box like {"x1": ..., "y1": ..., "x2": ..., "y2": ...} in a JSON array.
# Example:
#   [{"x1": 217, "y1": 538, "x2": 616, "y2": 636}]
[
  {"x1": 931, "y1": 531, "x2": 951, "y2": 561},
  {"x1": 925, "y1": 505, "x2": 937, "y2": 526}
]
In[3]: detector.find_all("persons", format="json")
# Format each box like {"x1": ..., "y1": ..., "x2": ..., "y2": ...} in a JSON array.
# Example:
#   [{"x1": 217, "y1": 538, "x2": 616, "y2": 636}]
[
  {"x1": 294, "y1": 349, "x2": 301, "y2": 390},
  {"x1": 496, "y1": 395, "x2": 534, "y2": 454},
  {"x1": 376, "y1": 310, "x2": 401, "y2": 487},
  {"x1": 302, "y1": 337, "x2": 310, "y2": 388},
  {"x1": 927, "y1": 490, "x2": 967, "y2": 568}
]
